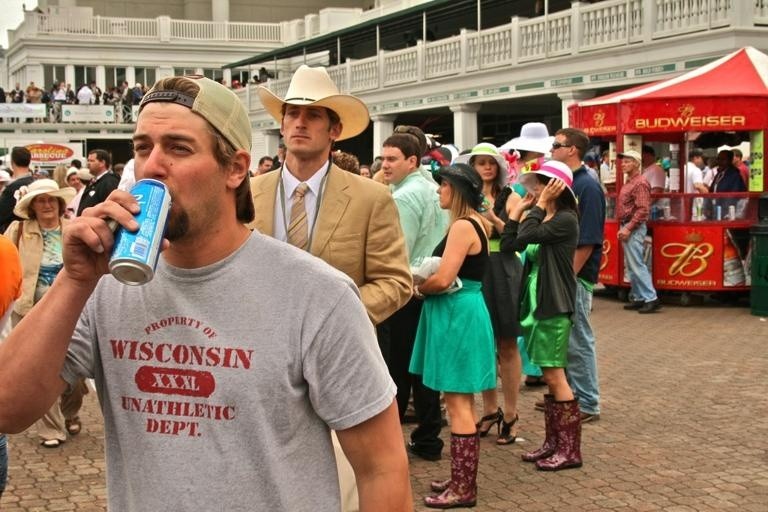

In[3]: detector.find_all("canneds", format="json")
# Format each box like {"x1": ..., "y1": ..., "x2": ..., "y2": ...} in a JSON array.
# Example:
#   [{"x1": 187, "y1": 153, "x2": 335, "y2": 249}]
[
  {"x1": 728, "y1": 205, "x2": 736, "y2": 222},
  {"x1": 650, "y1": 204, "x2": 658, "y2": 221},
  {"x1": 108, "y1": 178, "x2": 173, "y2": 287},
  {"x1": 714, "y1": 204, "x2": 723, "y2": 222}
]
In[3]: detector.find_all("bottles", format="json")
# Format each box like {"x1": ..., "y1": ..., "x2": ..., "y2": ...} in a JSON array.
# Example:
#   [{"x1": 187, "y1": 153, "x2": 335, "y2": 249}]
[
  {"x1": 723, "y1": 227, "x2": 745, "y2": 287},
  {"x1": 744, "y1": 240, "x2": 752, "y2": 277}
]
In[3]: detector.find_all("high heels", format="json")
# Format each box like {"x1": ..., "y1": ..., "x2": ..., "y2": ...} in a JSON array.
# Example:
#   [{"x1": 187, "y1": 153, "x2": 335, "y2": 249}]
[
  {"x1": 475, "y1": 407, "x2": 504, "y2": 438},
  {"x1": 496, "y1": 414, "x2": 518, "y2": 445}
]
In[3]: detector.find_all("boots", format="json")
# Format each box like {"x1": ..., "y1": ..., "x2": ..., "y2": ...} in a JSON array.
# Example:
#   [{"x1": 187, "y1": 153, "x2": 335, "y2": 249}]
[
  {"x1": 429, "y1": 433, "x2": 480, "y2": 494},
  {"x1": 521, "y1": 394, "x2": 557, "y2": 462},
  {"x1": 535, "y1": 396, "x2": 582, "y2": 470},
  {"x1": 424, "y1": 433, "x2": 478, "y2": 509}
]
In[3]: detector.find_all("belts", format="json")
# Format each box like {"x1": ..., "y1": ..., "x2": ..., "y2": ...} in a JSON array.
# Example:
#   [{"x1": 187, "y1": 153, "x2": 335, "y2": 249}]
[{"x1": 620, "y1": 220, "x2": 629, "y2": 225}]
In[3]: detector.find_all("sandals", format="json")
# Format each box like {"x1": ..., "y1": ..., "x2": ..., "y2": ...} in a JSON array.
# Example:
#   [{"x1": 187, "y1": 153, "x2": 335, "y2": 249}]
[
  {"x1": 524, "y1": 375, "x2": 547, "y2": 387},
  {"x1": 64, "y1": 417, "x2": 81, "y2": 435},
  {"x1": 42, "y1": 439, "x2": 60, "y2": 448}
]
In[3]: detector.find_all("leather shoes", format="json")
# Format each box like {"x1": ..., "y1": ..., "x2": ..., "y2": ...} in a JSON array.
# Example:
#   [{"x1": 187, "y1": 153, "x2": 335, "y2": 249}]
[
  {"x1": 623, "y1": 300, "x2": 644, "y2": 310},
  {"x1": 580, "y1": 410, "x2": 600, "y2": 423},
  {"x1": 638, "y1": 298, "x2": 664, "y2": 313},
  {"x1": 407, "y1": 441, "x2": 441, "y2": 462}
]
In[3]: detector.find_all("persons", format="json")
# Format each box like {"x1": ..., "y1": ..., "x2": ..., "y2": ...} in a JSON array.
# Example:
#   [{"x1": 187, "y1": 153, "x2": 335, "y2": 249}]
[
  {"x1": 2, "y1": 147, "x2": 135, "y2": 494},
  {"x1": 2, "y1": 81, "x2": 144, "y2": 124},
  {"x1": 229, "y1": 66, "x2": 271, "y2": 91},
  {"x1": 415, "y1": 163, "x2": 499, "y2": 507},
  {"x1": 606, "y1": 138, "x2": 751, "y2": 314},
  {"x1": 334, "y1": 122, "x2": 605, "y2": 467},
  {"x1": 2, "y1": 74, "x2": 413, "y2": 511},
  {"x1": 256, "y1": 145, "x2": 287, "y2": 175},
  {"x1": 245, "y1": 65, "x2": 414, "y2": 511}
]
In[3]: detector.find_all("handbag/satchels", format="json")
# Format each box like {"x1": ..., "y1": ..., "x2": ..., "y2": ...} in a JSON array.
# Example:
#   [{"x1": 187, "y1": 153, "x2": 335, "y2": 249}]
[{"x1": 409, "y1": 256, "x2": 462, "y2": 296}]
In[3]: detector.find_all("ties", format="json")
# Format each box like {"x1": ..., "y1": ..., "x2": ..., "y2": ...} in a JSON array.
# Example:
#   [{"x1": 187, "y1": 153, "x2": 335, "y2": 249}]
[{"x1": 286, "y1": 182, "x2": 310, "y2": 251}]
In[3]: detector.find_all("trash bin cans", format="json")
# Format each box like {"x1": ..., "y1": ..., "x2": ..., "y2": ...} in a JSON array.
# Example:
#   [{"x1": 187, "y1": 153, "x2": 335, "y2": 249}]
[{"x1": 749, "y1": 222, "x2": 768, "y2": 317}]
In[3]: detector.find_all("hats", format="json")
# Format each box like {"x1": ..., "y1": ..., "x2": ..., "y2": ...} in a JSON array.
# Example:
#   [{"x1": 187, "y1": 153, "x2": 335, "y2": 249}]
[
  {"x1": 519, "y1": 158, "x2": 579, "y2": 209},
  {"x1": 14, "y1": 178, "x2": 76, "y2": 220},
  {"x1": 64, "y1": 166, "x2": 78, "y2": 186},
  {"x1": 452, "y1": 142, "x2": 508, "y2": 186},
  {"x1": 616, "y1": 150, "x2": 641, "y2": 166},
  {"x1": 136, "y1": 75, "x2": 255, "y2": 223},
  {"x1": 257, "y1": 64, "x2": 370, "y2": 143},
  {"x1": 433, "y1": 163, "x2": 483, "y2": 205},
  {"x1": 500, "y1": 122, "x2": 556, "y2": 153},
  {"x1": 76, "y1": 168, "x2": 95, "y2": 181}
]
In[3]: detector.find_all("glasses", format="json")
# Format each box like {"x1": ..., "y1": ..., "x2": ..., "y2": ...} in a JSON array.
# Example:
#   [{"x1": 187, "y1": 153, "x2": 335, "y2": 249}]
[
  {"x1": 553, "y1": 142, "x2": 572, "y2": 148},
  {"x1": 394, "y1": 124, "x2": 420, "y2": 140}
]
